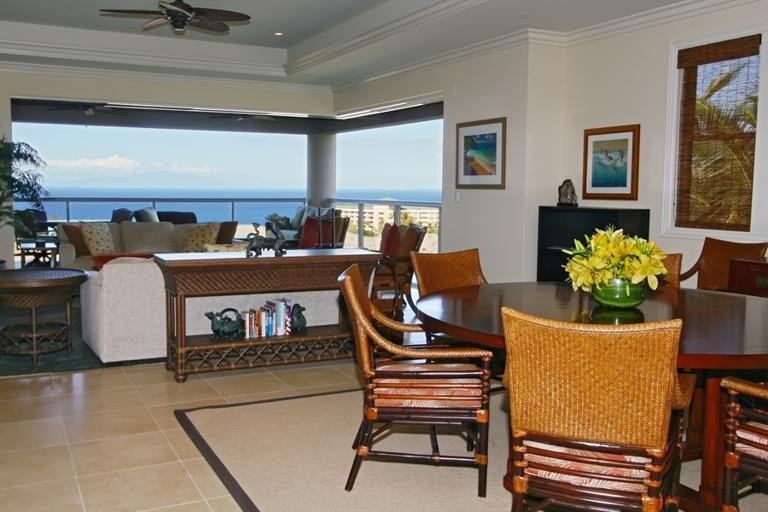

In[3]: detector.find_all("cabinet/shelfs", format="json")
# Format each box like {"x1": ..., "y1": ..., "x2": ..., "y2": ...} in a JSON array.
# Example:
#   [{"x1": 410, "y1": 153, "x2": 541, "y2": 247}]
[{"x1": 152, "y1": 244, "x2": 407, "y2": 382}]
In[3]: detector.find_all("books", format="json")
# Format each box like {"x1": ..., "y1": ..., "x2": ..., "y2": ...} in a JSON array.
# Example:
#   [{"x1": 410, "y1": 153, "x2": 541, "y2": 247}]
[{"x1": 241, "y1": 298, "x2": 290, "y2": 340}]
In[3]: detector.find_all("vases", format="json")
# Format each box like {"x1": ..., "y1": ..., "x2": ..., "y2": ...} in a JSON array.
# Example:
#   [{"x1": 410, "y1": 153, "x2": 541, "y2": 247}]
[
  {"x1": 588, "y1": 304, "x2": 644, "y2": 324},
  {"x1": 588, "y1": 276, "x2": 645, "y2": 309}
]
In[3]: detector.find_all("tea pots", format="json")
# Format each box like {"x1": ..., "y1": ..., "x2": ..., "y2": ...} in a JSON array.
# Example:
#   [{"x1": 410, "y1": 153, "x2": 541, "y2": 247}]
[{"x1": 205, "y1": 308, "x2": 246, "y2": 341}]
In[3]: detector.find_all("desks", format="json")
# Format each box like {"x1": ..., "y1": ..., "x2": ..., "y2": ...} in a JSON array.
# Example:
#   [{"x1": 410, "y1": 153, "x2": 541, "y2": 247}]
[
  {"x1": 15, "y1": 234, "x2": 59, "y2": 268},
  {"x1": 0, "y1": 267, "x2": 87, "y2": 367},
  {"x1": 414, "y1": 280, "x2": 767, "y2": 490}
]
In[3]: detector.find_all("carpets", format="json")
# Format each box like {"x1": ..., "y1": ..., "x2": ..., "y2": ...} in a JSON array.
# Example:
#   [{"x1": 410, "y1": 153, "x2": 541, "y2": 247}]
[
  {"x1": 0, "y1": 294, "x2": 169, "y2": 382},
  {"x1": 173, "y1": 386, "x2": 768, "y2": 512}
]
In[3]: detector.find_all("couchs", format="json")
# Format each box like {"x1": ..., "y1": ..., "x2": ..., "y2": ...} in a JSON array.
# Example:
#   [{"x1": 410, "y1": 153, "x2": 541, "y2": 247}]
[
  {"x1": 54, "y1": 222, "x2": 254, "y2": 270},
  {"x1": 266, "y1": 206, "x2": 341, "y2": 243},
  {"x1": 78, "y1": 252, "x2": 339, "y2": 364}
]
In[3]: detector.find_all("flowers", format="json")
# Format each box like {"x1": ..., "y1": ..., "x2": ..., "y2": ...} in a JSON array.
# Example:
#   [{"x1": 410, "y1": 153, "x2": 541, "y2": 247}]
[{"x1": 563, "y1": 224, "x2": 667, "y2": 291}]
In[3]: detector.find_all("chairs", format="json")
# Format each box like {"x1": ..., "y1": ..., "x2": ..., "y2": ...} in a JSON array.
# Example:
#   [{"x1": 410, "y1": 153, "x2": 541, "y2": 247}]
[
  {"x1": 498, "y1": 304, "x2": 683, "y2": 512},
  {"x1": 157, "y1": 210, "x2": 197, "y2": 224},
  {"x1": 717, "y1": 366, "x2": 767, "y2": 512},
  {"x1": 134, "y1": 206, "x2": 159, "y2": 221},
  {"x1": 678, "y1": 237, "x2": 767, "y2": 291},
  {"x1": 110, "y1": 208, "x2": 134, "y2": 223},
  {"x1": 408, "y1": 248, "x2": 507, "y2": 395},
  {"x1": 14, "y1": 209, "x2": 49, "y2": 265},
  {"x1": 658, "y1": 253, "x2": 682, "y2": 288},
  {"x1": 338, "y1": 264, "x2": 493, "y2": 500},
  {"x1": 375, "y1": 224, "x2": 427, "y2": 317}
]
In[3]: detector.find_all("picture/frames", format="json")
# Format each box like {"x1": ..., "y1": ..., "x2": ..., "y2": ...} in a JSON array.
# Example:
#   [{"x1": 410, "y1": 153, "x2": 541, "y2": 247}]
[
  {"x1": 583, "y1": 124, "x2": 637, "y2": 199},
  {"x1": 455, "y1": 117, "x2": 506, "y2": 190}
]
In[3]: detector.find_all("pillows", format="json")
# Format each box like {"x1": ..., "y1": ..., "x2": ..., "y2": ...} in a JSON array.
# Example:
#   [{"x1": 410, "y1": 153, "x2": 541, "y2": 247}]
[
  {"x1": 63, "y1": 221, "x2": 240, "y2": 254},
  {"x1": 290, "y1": 207, "x2": 333, "y2": 229}
]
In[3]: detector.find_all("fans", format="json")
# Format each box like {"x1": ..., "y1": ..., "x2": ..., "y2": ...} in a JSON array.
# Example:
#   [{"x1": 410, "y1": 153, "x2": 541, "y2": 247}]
[{"x1": 98, "y1": 0, "x2": 251, "y2": 34}]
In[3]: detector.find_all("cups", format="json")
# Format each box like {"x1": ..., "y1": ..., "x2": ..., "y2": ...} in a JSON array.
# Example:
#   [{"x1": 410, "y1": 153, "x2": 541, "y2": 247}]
[{"x1": 35, "y1": 239, "x2": 46, "y2": 248}]
